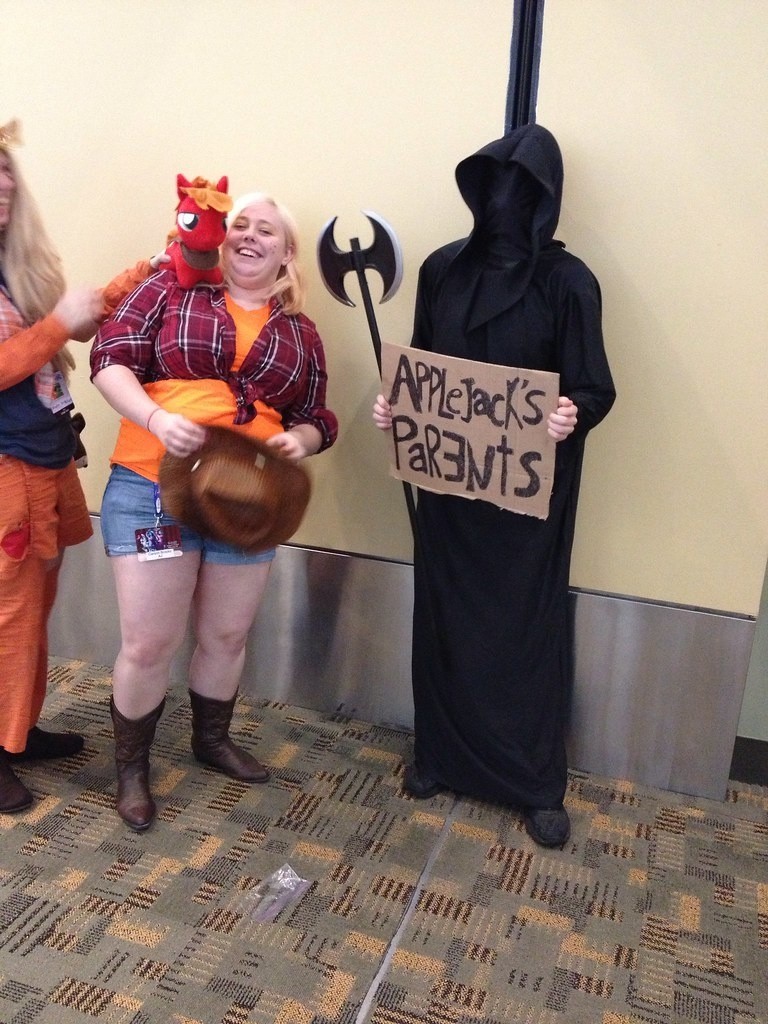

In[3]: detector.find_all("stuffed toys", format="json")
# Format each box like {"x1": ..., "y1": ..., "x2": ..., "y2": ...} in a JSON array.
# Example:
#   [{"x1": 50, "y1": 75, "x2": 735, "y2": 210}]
[{"x1": 156, "y1": 173, "x2": 234, "y2": 292}]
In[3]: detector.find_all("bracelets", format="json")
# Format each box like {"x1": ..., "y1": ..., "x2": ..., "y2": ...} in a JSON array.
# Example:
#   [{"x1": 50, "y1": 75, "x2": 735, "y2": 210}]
[{"x1": 147, "y1": 408, "x2": 162, "y2": 432}]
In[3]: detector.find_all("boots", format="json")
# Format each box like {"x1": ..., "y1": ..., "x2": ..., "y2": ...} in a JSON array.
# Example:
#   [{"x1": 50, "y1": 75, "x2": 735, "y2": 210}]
[
  {"x1": 110, "y1": 692, "x2": 168, "y2": 829},
  {"x1": 188, "y1": 685, "x2": 271, "y2": 782}
]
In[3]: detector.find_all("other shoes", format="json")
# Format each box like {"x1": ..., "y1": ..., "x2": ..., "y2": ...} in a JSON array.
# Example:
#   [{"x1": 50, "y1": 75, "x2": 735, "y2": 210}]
[
  {"x1": 0, "y1": 726, "x2": 84, "y2": 762},
  {"x1": 405, "y1": 762, "x2": 444, "y2": 798},
  {"x1": 523, "y1": 804, "x2": 570, "y2": 847},
  {"x1": 0, "y1": 747, "x2": 32, "y2": 812}
]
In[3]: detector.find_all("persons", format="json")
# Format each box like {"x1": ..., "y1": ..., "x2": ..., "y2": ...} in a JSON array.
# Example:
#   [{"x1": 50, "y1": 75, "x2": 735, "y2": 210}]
[
  {"x1": 90, "y1": 192, "x2": 338, "y2": 830},
  {"x1": 371, "y1": 122, "x2": 618, "y2": 850},
  {"x1": 0, "y1": 130, "x2": 177, "y2": 815}
]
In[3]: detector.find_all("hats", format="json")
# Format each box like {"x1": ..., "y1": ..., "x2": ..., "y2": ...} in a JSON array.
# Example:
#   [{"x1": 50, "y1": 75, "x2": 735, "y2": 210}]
[{"x1": 159, "y1": 423, "x2": 310, "y2": 553}]
